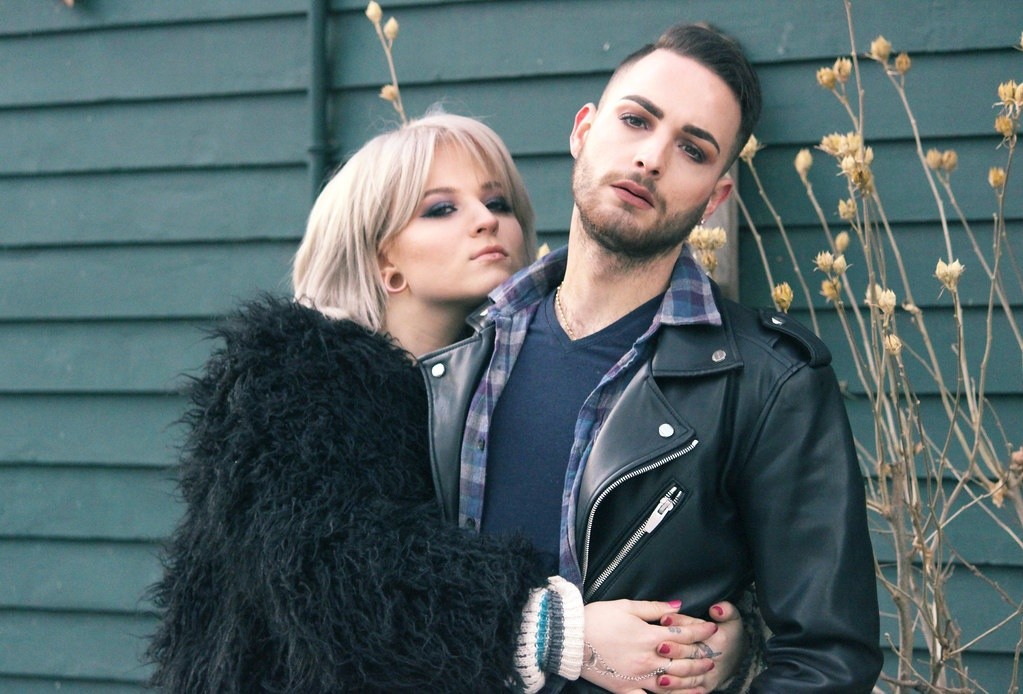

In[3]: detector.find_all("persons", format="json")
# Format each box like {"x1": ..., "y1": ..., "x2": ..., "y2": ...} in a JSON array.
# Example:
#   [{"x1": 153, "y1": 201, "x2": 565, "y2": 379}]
[
  {"x1": 414, "y1": 20, "x2": 888, "y2": 694},
  {"x1": 139, "y1": 109, "x2": 747, "y2": 693}
]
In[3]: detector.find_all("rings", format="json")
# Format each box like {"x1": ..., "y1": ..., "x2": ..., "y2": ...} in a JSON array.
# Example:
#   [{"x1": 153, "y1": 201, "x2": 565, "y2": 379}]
[{"x1": 582, "y1": 640, "x2": 673, "y2": 681}]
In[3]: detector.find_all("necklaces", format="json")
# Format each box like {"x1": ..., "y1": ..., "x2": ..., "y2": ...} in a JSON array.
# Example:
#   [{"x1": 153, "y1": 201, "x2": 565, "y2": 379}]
[{"x1": 557, "y1": 281, "x2": 579, "y2": 343}]
